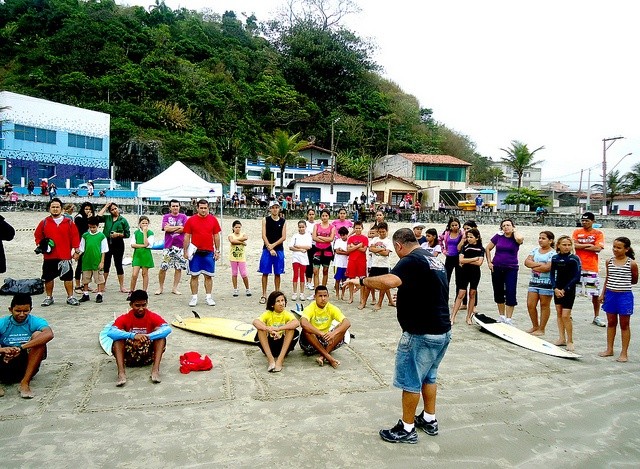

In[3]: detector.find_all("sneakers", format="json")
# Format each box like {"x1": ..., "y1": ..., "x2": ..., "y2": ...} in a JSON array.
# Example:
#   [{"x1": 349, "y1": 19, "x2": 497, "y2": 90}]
[
  {"x1": 496, "y1": 315, "x2": 505, "y2": 322},
  {"x1": 119, "y1": 287, "x2": 128, "y2": 292},
  {"x1": 127, "y1": 290, "x2": 136, "y2": 300},
  {"x1": 246, "y1": 289, "x2": 252, "y2": 295},
  {"x1": 96, "y1": 294, "x2": 102, "y2": 303},
  {"x1": 414, "y1": 410, "x2": 438, "y2": 435},
  {"x1": 81, "y1": 284, "x2": 93, "y2": 290},
  {"x1": 41, "y1": 298, "x2": 55, "y2": 306},
  {"x1": 66, "y1": 297, "x2": 80, "y2": 305},
  {"x1": 379, "y1": 419, "x2": 418, "y2": 444},
  {"x1": 299, "y1": 293, "x2": 306, "y2": 300},
  {"x1": 593, "y1": 315, "x2": 606, "y2": 326},
  {"x1": 307, "y1": 294, "x2": 315, "y2": 299},
  {"x1": 92, "y1": 287, "x2": 104, "y2": 292},
  {"x1": 75, "y1": 286, "x2": 84, "y2": 294},
  {"x1": 305, "y1": 283, "x2": 314, "y2": 289},
  {"x1": 189, "y1": 297, "x2": 198, "y2": 306},
  {"x1": 205, "y1": 298, "x2": 216, "y2": 306},
  {"x1": 291, "y1": 294, "x2": 297, "y2": 301},
  {"x1": 233, "y1": 290, "x2": 239, "y2": 296},
  {"x1": 79, "y1": 294, "x2": 89, "y2": 302},
  {"x1": 505, "y1": 318, "x2": 512, "y2": 325}
]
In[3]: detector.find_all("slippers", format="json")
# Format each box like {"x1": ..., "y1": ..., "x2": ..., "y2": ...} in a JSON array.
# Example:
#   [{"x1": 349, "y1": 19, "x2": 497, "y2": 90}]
[{"x1": 259, "y1": 296, "x2": 266, "y2": 303}]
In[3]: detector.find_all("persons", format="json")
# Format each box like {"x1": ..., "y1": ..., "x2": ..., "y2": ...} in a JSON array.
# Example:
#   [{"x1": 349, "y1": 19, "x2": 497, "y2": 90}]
[
  {"x1": 73, "y1": 215, "x2": 109, "y2": 303},
  {"x1": 402, "y1": 192, "x2": 413, "y2": 210},
  {"x1": 414, "y1": 199, "x2": 421, "y2": 213},
  {"x1": 74, "y1": 202, "x2": 95, "y2": 294},
  {"x1": 367, "y1": 224, "x2": 397, "y2": 307},
  {"x1": 246, "y1": 192, "x2": 252, "y2": 205},
  {"x1": 0, "y1": 175, "x2": 10, "y2": 196},
  {"x1": 360, "y1": 192, "x2": 367, "y2": 204},
  {"x1": 0, "y1": 215, "x2": 15, "y2": 273},
  {"x1": 571, "y1": 212, "x2": 606, "y2": 327},
  {"x1": 252, "y1": 291, "x2": 301, "y2": 372},
  {"x1": 40, "y1": 178, "x2": 48, "y2": 195},
  {"x1": 228, "y1": 220, "x2": 251, "y2": 296},
  {"x1": 87, "y1": 180, "x2": 94, "y2": 199},
  {"x1": 333, "y1": 226, "x2": 349, "y2": 300},
  {"x1": 222, "y1": 194, "x2": 227, "y2": 215},
  {"x1": 155, "y1": 199, "x2": 188, "y2": 295},
  {"x1": 377, "y1": 200, "x2": 385, "y2": 213},
  {"x1": 4, "y1": 181, "x2": 14, "y2": 196},
  {"x1": 439, "y1": 199, "x2": 446, "y2": 213},
  {"x1": 523, "y1": 231, "x2": 557, "y2": 336},
  {"x1": 420, "y1": 228, "x2": 441, "y2": 259},
  {"x1": 307, "y1": 209, "x2": 336, "y2": 301},
  {"x1": 226, "y1": 190, "x2": 231, "y2": 207},
  {"x1": 347, "y1": 221, "x2": 369, "y2": 305},
  {"x1": 358, "y1": 222, "x2": 394, "y2": 311},
  {"x1": 475, "y1": 195, "x2": 483, "y2": 213},
  {"x1": 332, "y1": 209, "x2": 353, "y2": 291},
  {"x1": 285, "y1": 195, "x2": 295, "y2": 211},
  {"x1": 352, "y1": 197, "x2": 359, "y2": 222},
  {"x1": 64, "y1": 204, "x2": 75, "y2": 221},
  {"x1": 369, "y1": 209, "x2": 385, "y2": 228},
  {"x1": 34, "y1": 198, "x2": 80, "y2": 306},
  {"x1": 536, "y1": 203, "x2": 544, "y2": 217},
  {"x1": 48, "y1": 182, "x2": 57, "y2": 200},
  {"x1": 92, "y1": 200, "x2": 130, "y2": 294},
  {"x1": 232, "y1": 192, "x2": 239, "y2": 209},
  {"x1": 239, "y1": 192, "x2": 247, "y2": 205},
  {"x1": 412, "y1": 222, "x2": 428, "y2": 246},
  {"x1": 300, "y1": 285, "x2": 352, "y2": 369},
  {"x1": 385, "y1": 203, "x2": 394, "y2": 222},
  {"x1": 268, "y1": 194, "x2": 276, "y2": 206},
  {"x1": 597, "y1": 237, "x2": 639, "y2": 362},
  {"x1": 107, "y1": 289, "x2": 172, "y2": 387},
  {"x1": 549, "y1": 234, "x2": 581, "y2": 350},
  {"x1": 277, "y1": 192, "x2": 284, "y2": 202},
  {"x1": 360, "y1": 202, "x2": 367, "y2": 223},
  {"x1": 486, "y1": 219, "x2": 524, "y2": 325},
  {"x1": 127, "y1": 215, "x2": 155, "y2": 301},
  {"x1": 183, "y1": 199, "x2": 222, "y2": 306},
  {"x1": 370, "y1": 190, "x2": 377, "y2": 204},
  {"x1": 289, "y1": 220, "x2": 313, "y2": 301},
  {"x1": 259, "y1": 192, "x2": 267, "y2": 208},
  {"x1": 450, "y1": 229, "x2": 485, "y2": 325},
  {"x1": 0, "y1": 293, "x2": 54, "y2": 399},
  {"x1": 409, "y1": 211, "x2": 418, "y2": 222},
  {"x1": 257, "y1": 201, "x2": 287, "y2": 304},
  {"x1": 341, "y1": 228, "x2": 452, "y2": 444},
  {"x1": 27, "y1": 179, "x2": 35, "y2": 195},
  {"x1": 279, "y1": 198, "x2": 287, "y2": 219},
  {"x1": 457, "y1": 220, "x2": 478, "y2": 313},
  {"x1": 292, "y1": 194, "x2": 302, "y2": 210},
  {"x1": 305, "y1": 210, "x2": 317, "y2": 290},
  {"x1": 369, "y1": 202, "x2": 376, "y2": 219},
  {"x1": 439, "y1": 217, "x2": 463, "y2": 308}
]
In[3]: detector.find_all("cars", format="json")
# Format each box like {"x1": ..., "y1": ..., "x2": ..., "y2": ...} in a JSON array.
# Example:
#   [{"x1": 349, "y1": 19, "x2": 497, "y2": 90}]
[{"x1": 79, "y1": 178, "x2": 118, "y2": 191}]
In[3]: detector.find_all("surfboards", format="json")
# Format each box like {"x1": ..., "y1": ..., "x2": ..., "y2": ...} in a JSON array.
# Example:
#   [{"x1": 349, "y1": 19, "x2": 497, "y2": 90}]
[
  {"x1": 171, "y1": 310, "x2": 260, "y2": 343},
  {"x1": 98, "y1": 318, "x2": 116, "y2": 356},
  {"x1": 290, "y1": 303, "x2": 350, "y2": 346},
  {"x1": 472, "y1": 313, "x2": 583, "y2": 358}
]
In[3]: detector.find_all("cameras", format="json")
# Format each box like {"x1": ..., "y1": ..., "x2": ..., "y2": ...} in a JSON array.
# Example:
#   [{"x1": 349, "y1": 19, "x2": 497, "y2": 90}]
[{"x1": 34, "y1": 246, "x2": 45, "y2": 255}]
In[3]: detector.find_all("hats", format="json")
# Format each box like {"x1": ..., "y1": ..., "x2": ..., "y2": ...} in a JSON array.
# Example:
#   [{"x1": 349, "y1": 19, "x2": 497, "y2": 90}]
[
  {"x1": 581, "y1": 211, "x2": 597, "y2": 222},
  {"x1": 413, "y1": 222, "x2": 426, "y2": 229},
  {"x1": 268, "y1": 200, "x2": 280, "y2": 208}
]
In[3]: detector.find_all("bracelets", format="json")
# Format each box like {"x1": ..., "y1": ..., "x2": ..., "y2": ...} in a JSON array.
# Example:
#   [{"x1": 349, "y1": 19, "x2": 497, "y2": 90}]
[
  {"x1": 215, "y1": 250, "x2": 220, "y2": 253},
  {"x1": 75, "y1": 249, "x2": 80, "y2": 254},
  {"x1": 359, "y1": 276, "x2": 366, "y2": 286},
  {"x1": 19, "y1": 345, "x2": 22, "y2": 350}
]
